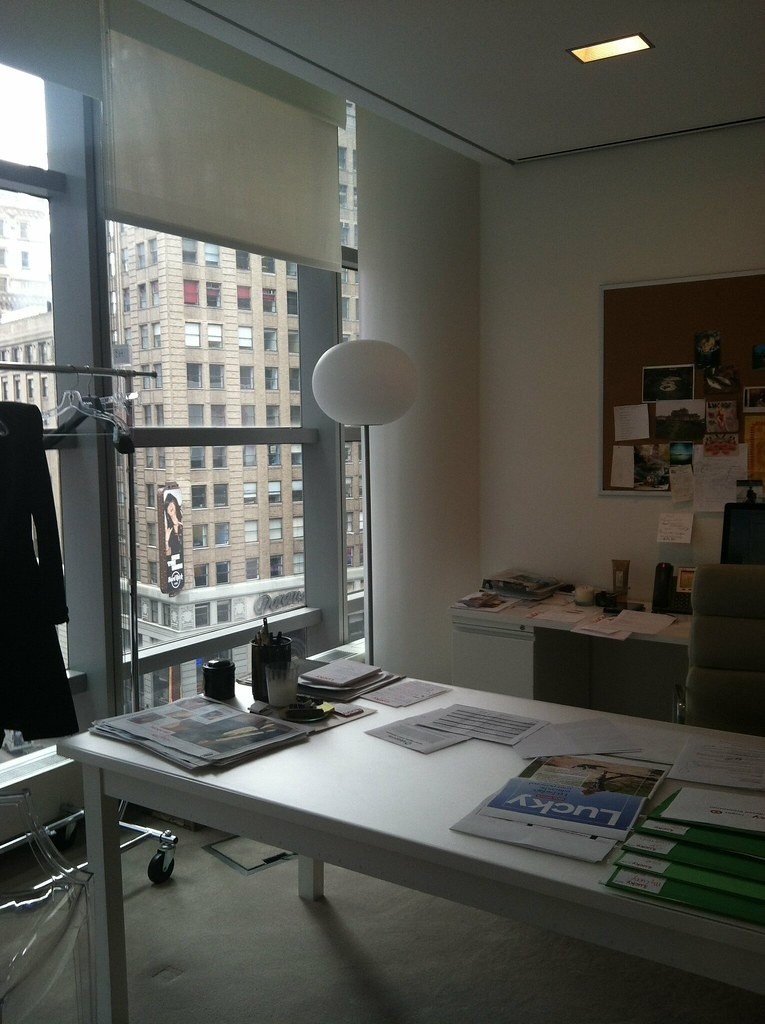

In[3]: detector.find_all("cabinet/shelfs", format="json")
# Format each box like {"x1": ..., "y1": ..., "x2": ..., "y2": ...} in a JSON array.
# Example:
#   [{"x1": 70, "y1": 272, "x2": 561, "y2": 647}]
[
  {"x1": 450, "y1": 624, "x2": 590, "y2": 708},
  {"x1": 451, "y1": 590, "x2": 692, "y2": 711}
]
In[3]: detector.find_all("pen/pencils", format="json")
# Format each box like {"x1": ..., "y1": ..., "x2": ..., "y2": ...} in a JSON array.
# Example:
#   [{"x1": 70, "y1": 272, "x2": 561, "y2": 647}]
[{"x1": 255, "y1": 617, "x2": 283, "y2": 645}]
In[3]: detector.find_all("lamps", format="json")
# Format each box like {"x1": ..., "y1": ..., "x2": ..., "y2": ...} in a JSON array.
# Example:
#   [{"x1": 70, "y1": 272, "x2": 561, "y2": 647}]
[{"x1": 314, "y1": 338, "x2": 417, "y2": 669}]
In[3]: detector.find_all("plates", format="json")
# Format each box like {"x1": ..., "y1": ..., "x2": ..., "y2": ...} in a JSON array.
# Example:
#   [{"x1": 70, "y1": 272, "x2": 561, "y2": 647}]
[{"x1": 279, "y1": 702, "x2": 328, "y2": 722}]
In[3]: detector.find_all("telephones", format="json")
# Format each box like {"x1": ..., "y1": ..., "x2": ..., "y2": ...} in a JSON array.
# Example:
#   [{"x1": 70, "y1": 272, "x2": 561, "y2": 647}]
[{"x1": 651, "y1": 562, "x2": 697, "y2": 616}]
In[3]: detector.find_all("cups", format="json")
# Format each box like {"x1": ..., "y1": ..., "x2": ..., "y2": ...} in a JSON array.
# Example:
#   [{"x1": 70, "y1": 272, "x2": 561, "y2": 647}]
[
  {"x1": 250, "y1": 637, "x2": 292, "y2": 703},
  {"x1": 265, "y1": 662, "x2": 299, "y2": 710},
  {"x1": 203, "y1": 659, "x2": 235, "y2": 700},
  {"x1": 575, "y1": 583, "x2": 594, "y2": 607}
]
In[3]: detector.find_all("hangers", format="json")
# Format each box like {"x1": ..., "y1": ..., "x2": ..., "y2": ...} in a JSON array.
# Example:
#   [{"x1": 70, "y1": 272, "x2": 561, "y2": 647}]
[{"x1": 38, "y1": 359, "x2": 139, "y2": 455}]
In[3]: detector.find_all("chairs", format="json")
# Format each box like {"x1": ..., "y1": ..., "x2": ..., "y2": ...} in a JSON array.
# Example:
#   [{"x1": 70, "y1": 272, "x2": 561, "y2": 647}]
[{"x1": 675, "y1": 564, "x2": 765, "y2": 737}]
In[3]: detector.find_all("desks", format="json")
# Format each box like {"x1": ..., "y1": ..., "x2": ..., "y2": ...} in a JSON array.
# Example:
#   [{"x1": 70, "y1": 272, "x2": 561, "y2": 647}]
[{"x1": 57, "y1": 656, "x2": 765, "y2": 1023}]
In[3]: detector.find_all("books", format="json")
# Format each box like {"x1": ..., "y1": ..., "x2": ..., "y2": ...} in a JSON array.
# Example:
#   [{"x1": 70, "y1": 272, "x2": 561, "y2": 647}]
[{"x1": 301, "y1": 659, "x2": 382, "y2": 686}]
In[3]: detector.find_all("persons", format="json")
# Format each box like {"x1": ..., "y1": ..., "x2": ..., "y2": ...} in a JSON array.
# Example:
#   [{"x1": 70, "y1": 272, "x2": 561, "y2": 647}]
[{"x1": 163, "y1": 494, "x2": 183, "y2": 557}]
